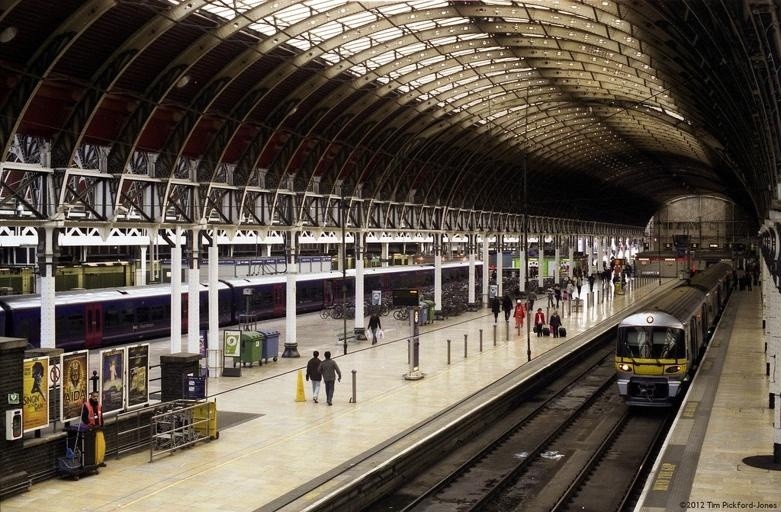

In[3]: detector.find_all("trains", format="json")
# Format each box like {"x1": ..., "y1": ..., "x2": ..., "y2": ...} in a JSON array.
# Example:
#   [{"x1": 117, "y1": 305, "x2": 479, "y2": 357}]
[{"x1": 614, "y1": 260, "x2": 735, "y2": 408}]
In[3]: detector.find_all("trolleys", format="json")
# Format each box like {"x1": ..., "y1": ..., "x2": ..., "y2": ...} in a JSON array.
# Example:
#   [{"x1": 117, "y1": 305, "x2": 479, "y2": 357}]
[
  {"x1": 57, "y1": 423, "x2": 98, "y2": 481},
  {"x1": 154, "y1": 405, "x2": 199, "y2": 450}
]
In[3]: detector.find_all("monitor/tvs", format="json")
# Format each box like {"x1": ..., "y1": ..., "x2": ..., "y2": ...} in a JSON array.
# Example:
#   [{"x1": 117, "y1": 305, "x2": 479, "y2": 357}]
[
  {"x1": 393, "y1": 289, "x2": 419, "y2": 306},
  {"x1": 613, "y1": 259, "x2": 625, "y2": 269}
]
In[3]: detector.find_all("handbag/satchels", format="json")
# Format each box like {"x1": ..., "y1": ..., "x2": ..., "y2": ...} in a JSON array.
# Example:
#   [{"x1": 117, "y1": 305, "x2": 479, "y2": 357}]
[
  {"x1": 534, "y1": 327, "x2": 537, "y2": 333},
  {"x1": 550, "y1": 326, "x2": 553, "y2": 332}
]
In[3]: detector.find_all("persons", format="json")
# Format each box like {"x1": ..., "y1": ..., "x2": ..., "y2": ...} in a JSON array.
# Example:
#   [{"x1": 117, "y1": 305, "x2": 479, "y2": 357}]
[
  {"x1": 306, "y1": 351, "x2": 322, "y2": 403},
  {"x1": 82, "y1": 391, "x2": 107, "y2": 467},
  {"x1": 317, "y1": 351, "x2": 341, "y2": 406},
  {"x1": 492, "y1": 257, "x2": 632, "y2": 338},
  {"x1": 733, "y1": 261, "x2": 760, "y2": 291},
  {"x1": 367, "y1": 311, "x2": 382, "y2": 345}
]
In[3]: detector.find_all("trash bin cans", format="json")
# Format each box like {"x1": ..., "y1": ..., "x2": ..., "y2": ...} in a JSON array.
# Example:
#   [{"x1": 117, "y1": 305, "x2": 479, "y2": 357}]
[
  {"x1": 407, "y1": 300, "x2": 435, "y2": 325},
  {"x1": 63, "y1": 424, "x2": 98, "y2": 480},
  {"x1": 234, "y1": 330, "x2": 280, "y2": 367}
]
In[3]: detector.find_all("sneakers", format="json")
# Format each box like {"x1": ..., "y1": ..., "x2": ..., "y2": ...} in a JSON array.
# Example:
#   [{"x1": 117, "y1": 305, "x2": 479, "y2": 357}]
[
  {"x1": 328, "y1": 400, "x2": 332, "y2": 405},
  {"x1": 314, "y1": 397, "x2": 318, "y2": 402}
]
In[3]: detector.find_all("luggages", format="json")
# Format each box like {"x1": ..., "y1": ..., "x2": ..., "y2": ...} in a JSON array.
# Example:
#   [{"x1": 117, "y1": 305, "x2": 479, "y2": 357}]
[
  {"x1": 559, "y1": 325, "x2": 566, "y2": 337},
  {"x1": 543, "y1": 324, "x2": 549, "y2": 336}
]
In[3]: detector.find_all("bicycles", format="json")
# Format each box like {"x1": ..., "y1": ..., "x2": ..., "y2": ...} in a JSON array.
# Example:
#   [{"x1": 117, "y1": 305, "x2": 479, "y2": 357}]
[
  {"x1": 393, "y1": 306, "x2": 409, "y2": 320},
  {"x1": 321, "y1": 297, "x2": 389, "y2": 320},
  {"x1": 441, "y1": 296, "x2": 468, "y2": 316}
]
